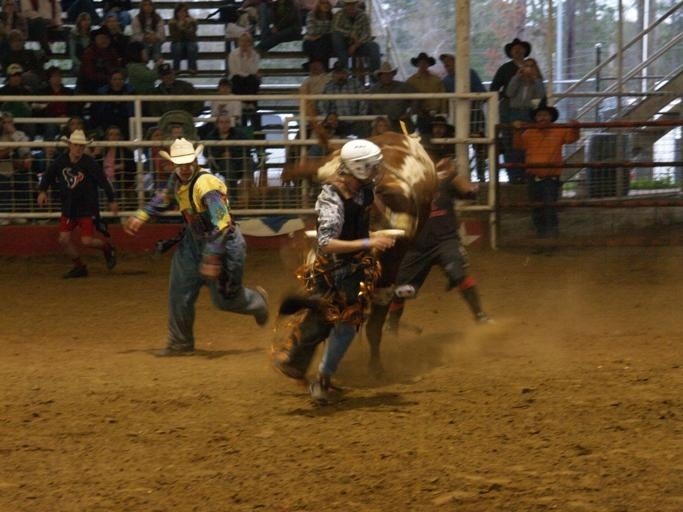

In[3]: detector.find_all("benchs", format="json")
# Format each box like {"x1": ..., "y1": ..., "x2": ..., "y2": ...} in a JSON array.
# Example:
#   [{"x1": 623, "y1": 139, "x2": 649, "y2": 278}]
[{"x1": 0, "y1": 0, "x2": 384, "y2": 168}]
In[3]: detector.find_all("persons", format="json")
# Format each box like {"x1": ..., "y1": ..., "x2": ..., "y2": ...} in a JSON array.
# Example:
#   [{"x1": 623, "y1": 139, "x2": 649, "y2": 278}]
[
  {"x1": 125, "y1": 139, "x2": 269, "y2": 356},
  {"x1": 1, "y1": 0, "x2": 488, "y2": 224},
  {"x1": 274, "y1": 132, "x2": 490, "y2": 403},
  {"x1": 512, "y1": 105, "x2": 580, "y2": 253},
  {"x1": 37, "y1": 129, "x2": 120, "y2": 279},
  {"x1": 490, "y1": 38, "x2": 547, "y2": 184}
]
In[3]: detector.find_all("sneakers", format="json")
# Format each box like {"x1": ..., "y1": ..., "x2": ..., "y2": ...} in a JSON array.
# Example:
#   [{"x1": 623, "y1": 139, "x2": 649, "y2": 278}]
[
  {"x1": 254, "y1": 285, "x2": 268, "y2": 326},
  {"x1": 104, "y1": 247, "x2": 117, "y2": 269},
  {"x1": 154, "y1": 348, "x2": 196, "y2": 358},
  {"x1": 63, "y1": 264, "x2": 88, "y2": 278}
]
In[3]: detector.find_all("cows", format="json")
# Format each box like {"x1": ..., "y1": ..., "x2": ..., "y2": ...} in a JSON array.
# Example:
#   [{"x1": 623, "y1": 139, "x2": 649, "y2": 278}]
[{"x1": 279, "y1": 120, "x2": 459, "y2": 379}]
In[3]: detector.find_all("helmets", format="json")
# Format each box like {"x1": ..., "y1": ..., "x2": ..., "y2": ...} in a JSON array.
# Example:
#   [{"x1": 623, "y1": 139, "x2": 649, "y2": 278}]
[{"x1": 340, "y1": 139, "x2": 385, "y2": 188}]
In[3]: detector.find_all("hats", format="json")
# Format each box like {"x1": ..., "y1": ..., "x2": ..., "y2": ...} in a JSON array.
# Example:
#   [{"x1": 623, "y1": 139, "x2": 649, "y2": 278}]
[
  {"x1": 158, "y1": 64, "x2": 173, "y2": 76},
  {"x1": 3, "y1": 63, "x2": 24, "y2": 82},
  {"x1": 60, "y1": 129, "x2": 94, "y2": 145},
  {"x1": 410, "y1": 53, "x2": 435, "y2": 67},
  {"x1": 323, "y1": 61, "x2": 355, "y2": 79},
  {"x1": 158, "y1": 138, "x2": 205, "y2": 164},
  {"x1": 373, "y1": 62, "x2": 398, "y2": 78},
  {"x1": 504, "y1": 38, "x2": 531, "y2": 58},
  {"x1": 425, "y1": 116, "x2": 454, "y2": 134},
  {"x1": 439, "y1": 54, "x2": 455, "y2": 62},
  {"x1": 529, "y1": 95, "x2": 558, "y2": 122}
]
